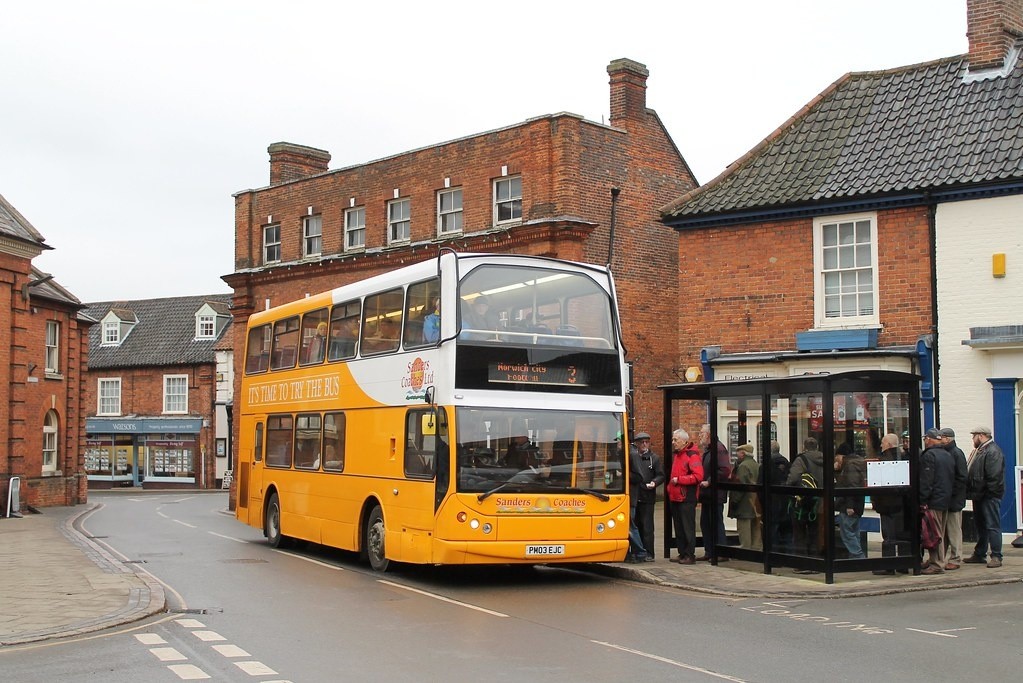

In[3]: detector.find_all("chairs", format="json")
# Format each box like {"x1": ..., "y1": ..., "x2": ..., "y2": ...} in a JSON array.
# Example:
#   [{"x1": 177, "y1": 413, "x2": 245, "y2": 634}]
[
  {"x1": 247, "y1": 320, "x2": 428, "y2": 371},
  {"x1": 526, "y1": 323, "x2": 553, "y2": 335},
  {"x1": 474, "y1": 446, "x2": 497, "y2": 468},
  {"x1": 556, "y1": 324, "x2": 581, "y2": 336}
]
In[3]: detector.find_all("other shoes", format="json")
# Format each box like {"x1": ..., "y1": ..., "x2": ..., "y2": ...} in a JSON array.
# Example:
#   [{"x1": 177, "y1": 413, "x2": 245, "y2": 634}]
[
  {"x1": 645, "y1": 556, "x2": 655, "y2": 562},
  {"x1": 872, "y1": 569, "x2": 910, "y2": 575},
  {"x1": 669, "y1": 554, "x2": 697, "y2": 564},
  {"x1": 626, "y1": 554, "x2": 647, "y2": 564},
  {"x1": 696, "y1": 556, "x2": 730, "y2": 563},
  {"x1": 920, "y1": 555, "x2": 1003, "y2": 575}
]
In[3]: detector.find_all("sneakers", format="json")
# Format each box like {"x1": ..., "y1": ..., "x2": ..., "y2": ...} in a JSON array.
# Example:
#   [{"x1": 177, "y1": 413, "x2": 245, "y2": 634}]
[{"x1": 792, "y1": 568, "x2": 818, "y2": 574}]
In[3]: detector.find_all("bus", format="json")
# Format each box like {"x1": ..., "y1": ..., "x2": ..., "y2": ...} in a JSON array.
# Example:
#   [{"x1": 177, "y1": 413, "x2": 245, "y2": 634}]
[{"x1": 234, "y1": 246, "x2": 630, "y2": 574}]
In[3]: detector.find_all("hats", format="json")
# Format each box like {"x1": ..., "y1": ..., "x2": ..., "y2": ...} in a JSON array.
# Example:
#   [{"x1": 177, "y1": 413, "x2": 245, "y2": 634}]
[
  {"x1": 736, "y1": 444, "x2": 753, "y2": 453},
  {"x1": 614, "y1": 434, "x2": 625, "y2": 441},
  {"x1": 317, "y1": 322, "x2": 327, "y2": 332},
  {"x1": 836, "y1": 442, "x2": 852, "y2": 454},
  {"x1": 634, "y1": 433, "x2": 650, "y2": 440},
  {"x1": 969, "y1": 425, "x2": 991, "y2": 433},
  {"x1": 381, "y1": 318, "x2": 392, "y2": 323},
  {"x1": 474, "y1": 296, "x2": 489, "y2": 307},
  {"x1": 901, "y1": 428, "x2": 955, "y2": 439}
]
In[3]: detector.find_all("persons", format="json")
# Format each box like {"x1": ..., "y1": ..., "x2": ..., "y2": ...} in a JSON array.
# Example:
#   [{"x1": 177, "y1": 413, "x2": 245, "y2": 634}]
[
  {"x1": 900, "y1": 431, "x2": 924, "y2": 562},
  {"x1": 695, "y1": 424, "x2": 732, "y2": 562},
  {"x1": 305, "y1": 294, "x2": 543, "y2": 364},
  {"x1": 547, "y1": 425, "x2": 584, "y2": 487},
  {"x1": 782, "y1": 437, "x2": 824, "y2": 575},
  {"x1": 605, "y1": 430, "x2": 649, "y2": 564},
  {"x1": 870, "y1": 433, "x2": 914, "y2": 575},
  {"x1": 727, "y1": 444, "x2": 763, "y2": 551},
  {"x1": 284, "y1": 430, "x2": 344, "y2": 472},
  {"x1": 757, "y1": 440, "x2": 796, "y2": 556},
  {"x1": 834, "y1": 441, "x2": 867, "y2": 560},
  {"x1": 917, "y1": 427, "x2": 955, "y2": 576},
  {"x1": 939, "y1": 428, "x2": 968, "y2": 570},
  {"x1": 962, "y1": 426, "x2": 1004, "y2": 568},
  {"x1": 667, "y1": 429, "x2": 704, "y2": 565},
  {"x1": 633, "y1": 432, "x2": 664, "y2": 562},
  {"x1": 503, "y1": 427, "x2": 538, "y2": 471}
]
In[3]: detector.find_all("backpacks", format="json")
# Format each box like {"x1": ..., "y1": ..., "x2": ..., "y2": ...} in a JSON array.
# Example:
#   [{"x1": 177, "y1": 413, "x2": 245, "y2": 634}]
[
  {"x1": 921, "y1": 506, "x2": 942, "y2": 550},
  {"x1": 792, "y1": 453, "x2": 817, "y2": 521}
]
